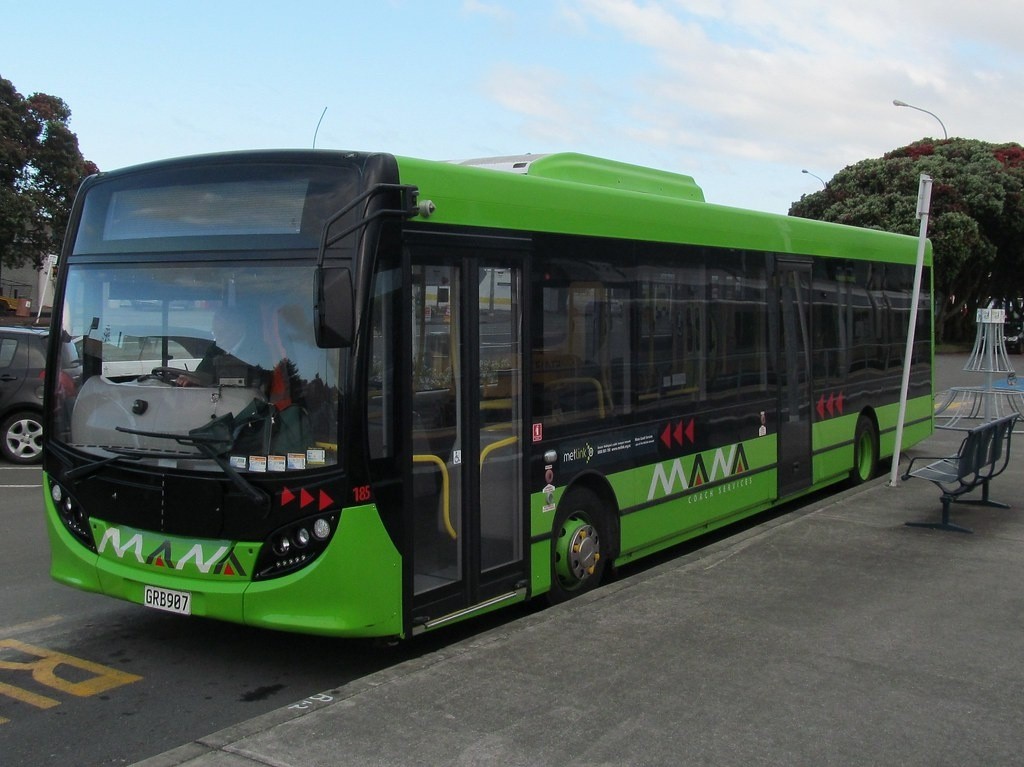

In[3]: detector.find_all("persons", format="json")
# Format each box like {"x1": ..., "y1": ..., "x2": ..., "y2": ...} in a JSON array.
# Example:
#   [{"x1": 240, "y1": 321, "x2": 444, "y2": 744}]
[{"x1": 177, "y1": 306, "x2": 270, "y2": 391}]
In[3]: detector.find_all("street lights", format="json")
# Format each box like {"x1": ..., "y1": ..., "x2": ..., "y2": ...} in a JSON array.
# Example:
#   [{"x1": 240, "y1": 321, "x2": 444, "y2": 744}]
[
  {"x1": 893, "y1": 98, "x2": 949, "y2": 142},
  {"x1": 800, "y1": 167, "x2": 826, "y2": 190}
]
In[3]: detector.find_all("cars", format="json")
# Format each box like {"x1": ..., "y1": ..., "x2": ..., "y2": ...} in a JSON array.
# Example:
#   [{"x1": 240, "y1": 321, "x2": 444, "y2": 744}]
[{"x1": 0, "y1": 323, "x2": 80, "y2": 467}]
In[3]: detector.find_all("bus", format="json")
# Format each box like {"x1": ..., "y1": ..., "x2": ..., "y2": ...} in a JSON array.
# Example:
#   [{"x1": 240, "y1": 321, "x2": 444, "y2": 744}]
[{"x1": 38, "y1": 152, "x2": 934, "y2": 646}]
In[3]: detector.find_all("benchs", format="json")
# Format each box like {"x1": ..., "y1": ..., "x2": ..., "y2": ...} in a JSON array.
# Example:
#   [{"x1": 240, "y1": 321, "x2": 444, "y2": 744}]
[{"x1": 902, "y1": 411, "x2": 1020, "y2": 536}]
